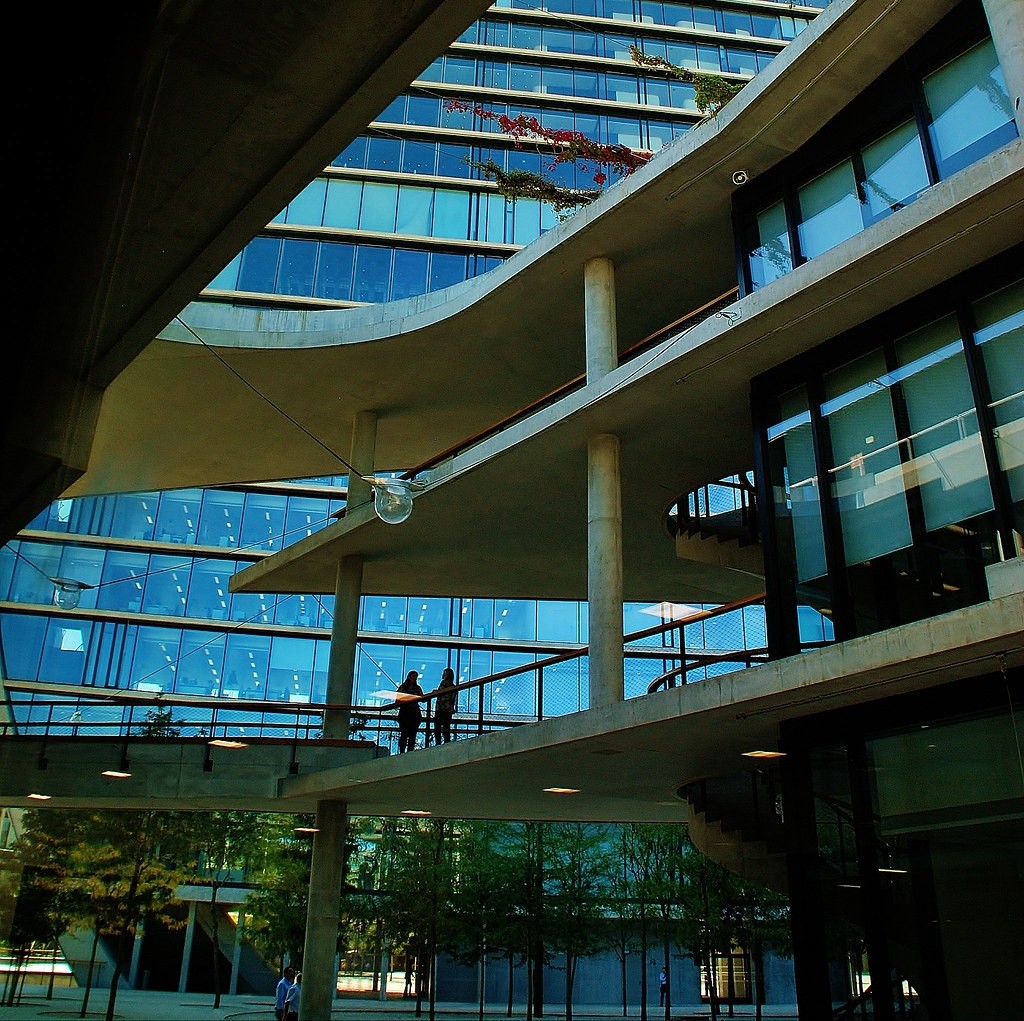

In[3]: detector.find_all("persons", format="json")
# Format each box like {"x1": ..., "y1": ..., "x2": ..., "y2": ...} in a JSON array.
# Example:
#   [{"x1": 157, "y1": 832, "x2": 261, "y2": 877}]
[
  {"x1": 395, "y1": 670, "x2": 424, "y2": 754},
  {"x1": 283, "y1": 973, "x2": 302, "y2": 1021},
  {"x1": 275, "y1": 968, "x2": 298, "y2": 1021},
  {"x1": 403, "y1": 959, "x2": 415, "y2": 997},
  {"x1": 432, "y1": 668, "x2": 458, "y2": 745},
  {"x1": 660, "y1": 967, "x2": 673, "y2": 1007}
]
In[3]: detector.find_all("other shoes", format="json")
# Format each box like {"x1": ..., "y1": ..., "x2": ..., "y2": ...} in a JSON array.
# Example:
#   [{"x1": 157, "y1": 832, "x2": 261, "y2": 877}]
[{"x1": 660, "y1": 1005, "x2": 663, "y2": 1007}]
